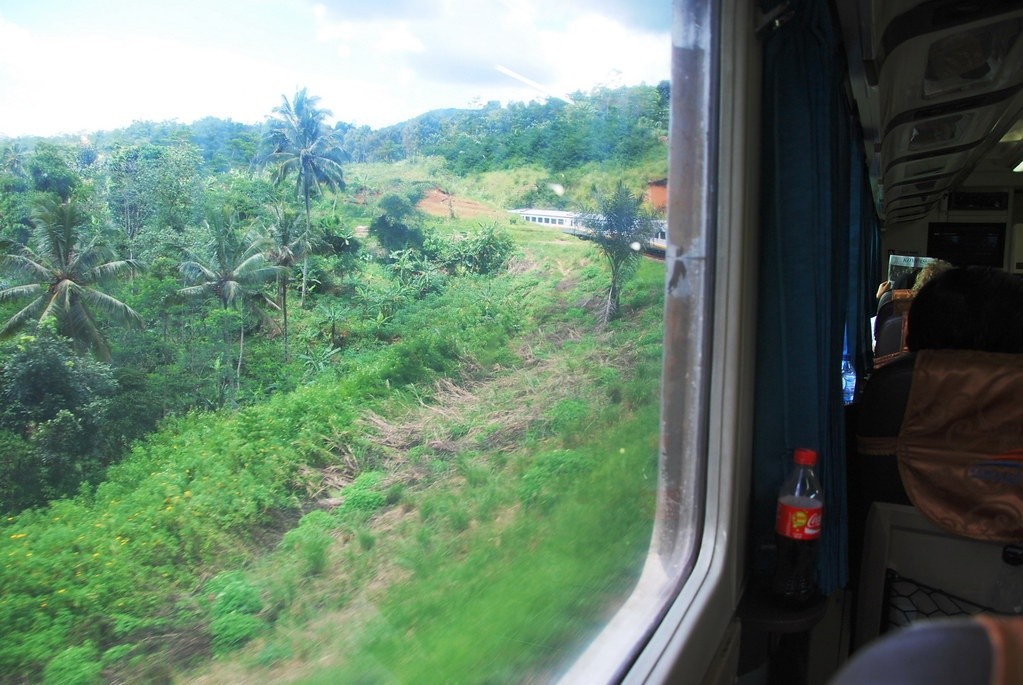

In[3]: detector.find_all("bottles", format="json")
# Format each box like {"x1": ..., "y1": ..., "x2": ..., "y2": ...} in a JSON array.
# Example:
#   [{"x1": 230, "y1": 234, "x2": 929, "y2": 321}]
[
  {"x1": 841, "y1": 354, "x2": 857, "y2": 406},
  {"x1": 774, "y1": 449, "x2": 825, "y2": 620}
]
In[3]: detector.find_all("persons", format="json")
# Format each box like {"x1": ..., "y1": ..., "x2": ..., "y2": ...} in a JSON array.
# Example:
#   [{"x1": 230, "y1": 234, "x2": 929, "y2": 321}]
[{"x1": 871, "y1": 258, "x2": 955, "y2": 314}]
